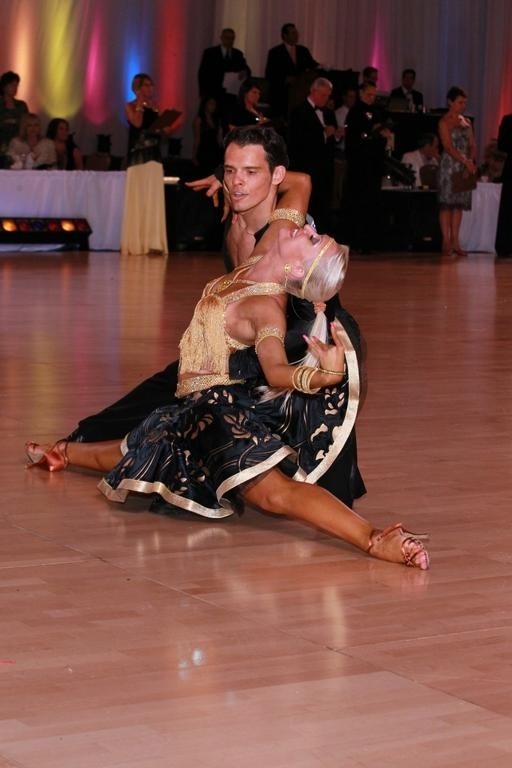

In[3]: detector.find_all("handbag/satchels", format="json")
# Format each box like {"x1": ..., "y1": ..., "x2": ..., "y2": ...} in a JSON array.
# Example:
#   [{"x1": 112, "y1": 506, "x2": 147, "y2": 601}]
[{"x1": 451, "y1": 170, "x2": 477, "y2": 193}]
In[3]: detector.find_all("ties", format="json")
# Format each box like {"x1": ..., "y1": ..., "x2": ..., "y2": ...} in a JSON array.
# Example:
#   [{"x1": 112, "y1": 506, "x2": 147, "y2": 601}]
[{"x1": 225, "y1": 49, "x2": 230, "y2": 60}]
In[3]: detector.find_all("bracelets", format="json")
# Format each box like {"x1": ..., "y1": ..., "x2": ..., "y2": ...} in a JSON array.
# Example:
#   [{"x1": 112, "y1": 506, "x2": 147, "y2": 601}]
[{"x1": 290, "y1": 365, "x2": 347, "y2": 396}]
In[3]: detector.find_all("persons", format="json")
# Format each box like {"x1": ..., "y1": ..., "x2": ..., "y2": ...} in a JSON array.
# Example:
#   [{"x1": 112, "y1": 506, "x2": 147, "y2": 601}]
[
  {"x1": 56, "y1": 124, "x2": 373, "y2": 512},
  {"x1": 3, "y1": 19, "x2": 483, "y2": 257},
  {"x1": 22, "y1": 164, "x2": 431, "y2": 572},
  {"x1": 493, "y1": 113, "x2": 511, "y2": 262}
]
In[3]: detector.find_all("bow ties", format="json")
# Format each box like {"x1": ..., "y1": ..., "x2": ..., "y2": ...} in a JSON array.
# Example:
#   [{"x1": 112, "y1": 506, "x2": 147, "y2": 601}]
[{"x1": 313, "y1": 105, "x2": 324, "y2": 112}]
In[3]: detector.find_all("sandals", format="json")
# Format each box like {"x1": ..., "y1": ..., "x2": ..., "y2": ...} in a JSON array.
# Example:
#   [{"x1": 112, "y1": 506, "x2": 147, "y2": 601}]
[{"x1": 444, "y1": 247, "x2": 467, "y2": 256}]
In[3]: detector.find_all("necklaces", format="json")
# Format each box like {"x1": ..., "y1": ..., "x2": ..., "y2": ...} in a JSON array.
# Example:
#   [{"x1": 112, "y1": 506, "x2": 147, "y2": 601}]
[{"x1": 215, "y1": 267, "x2": 257, "y2": 293}]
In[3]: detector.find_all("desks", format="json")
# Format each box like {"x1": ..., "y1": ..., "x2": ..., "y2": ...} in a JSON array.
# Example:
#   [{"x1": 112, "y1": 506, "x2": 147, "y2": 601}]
[
  {"x1": 0, "y1": 169, "x2": 127, "y2": 252},
  {"x1": 448, "y1": 182, "x2": 504, "y2": 255},
  {"x1": 374, "y1": 185, "x2": 441, "y2": 252},
  {"x1": 314, "y1": 66, "x2": 360, "y2": 97},
  {"x1": 247, "y1": 77, "x2": 264, "y2": 96}
]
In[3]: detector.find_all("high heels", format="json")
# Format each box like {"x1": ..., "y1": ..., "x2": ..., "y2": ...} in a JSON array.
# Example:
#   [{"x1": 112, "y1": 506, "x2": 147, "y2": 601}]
[
  {"x1": 367, "y1": 522, "x2": 432, "y2": 571},
  {"x1": 23, "y1": 440, "x2": 67, "y2": 472}
]
